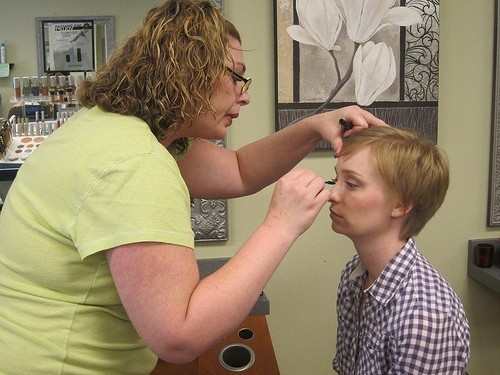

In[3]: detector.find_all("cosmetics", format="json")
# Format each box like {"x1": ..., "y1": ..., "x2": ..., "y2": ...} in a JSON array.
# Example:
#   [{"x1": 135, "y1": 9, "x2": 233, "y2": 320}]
[{"x1": 0, "y1": 71, "x2": 94, "y2": 163}]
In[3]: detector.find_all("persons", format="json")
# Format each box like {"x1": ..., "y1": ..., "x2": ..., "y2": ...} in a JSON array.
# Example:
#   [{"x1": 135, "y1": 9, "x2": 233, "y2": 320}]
[
  {"x1": 326, "y1": 126, "x2": 472, "y2": 375},
  {"x1": 0, "y1": 0, "x2": 391, "y2": 375}
]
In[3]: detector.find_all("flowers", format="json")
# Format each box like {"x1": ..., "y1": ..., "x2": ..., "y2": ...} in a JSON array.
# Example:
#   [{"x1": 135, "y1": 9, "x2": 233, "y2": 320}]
[{"x1": 287, "y1": 0, "x2": 422, "y2": 126}]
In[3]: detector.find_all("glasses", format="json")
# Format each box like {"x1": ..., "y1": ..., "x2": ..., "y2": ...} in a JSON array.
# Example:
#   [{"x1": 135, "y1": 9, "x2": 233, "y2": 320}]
[{"x1": 222, "y1": 63, "x2": 252, "y2": 94}]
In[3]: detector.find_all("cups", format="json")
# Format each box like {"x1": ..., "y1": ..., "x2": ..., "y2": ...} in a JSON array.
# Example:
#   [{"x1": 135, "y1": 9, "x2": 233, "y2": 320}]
[{"x1": 476, "y1": 243, "x2": 494, "y2": 268}]
